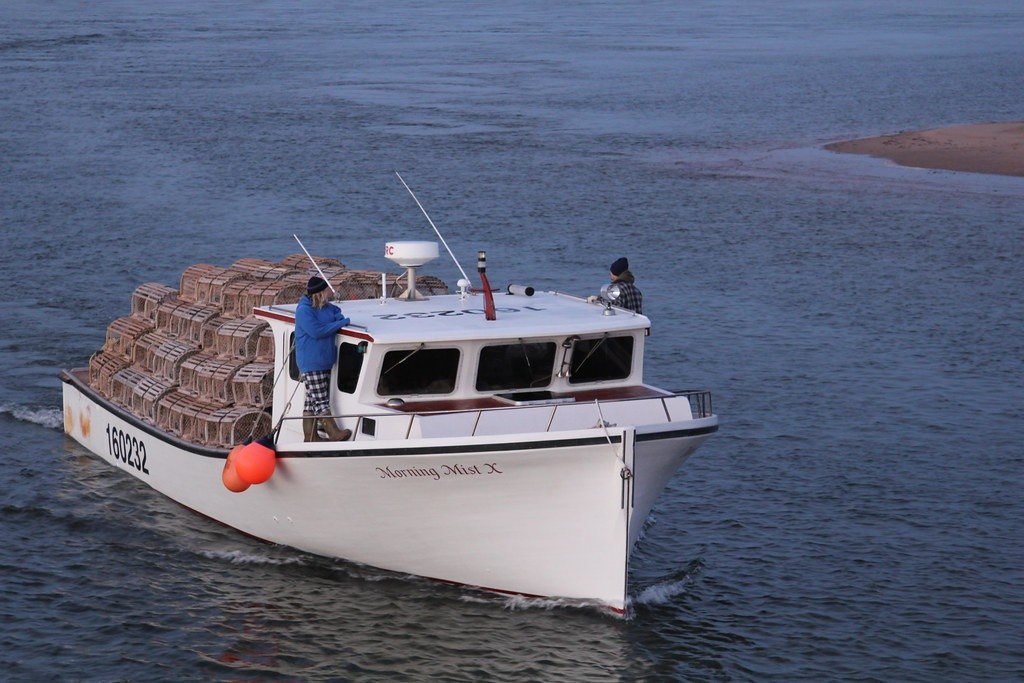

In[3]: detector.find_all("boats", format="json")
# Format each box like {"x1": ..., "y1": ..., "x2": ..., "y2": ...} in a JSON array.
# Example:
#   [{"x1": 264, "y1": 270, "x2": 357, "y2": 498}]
[{"x1": 56, "y1": 165, "x2": 720, "y2": 622}]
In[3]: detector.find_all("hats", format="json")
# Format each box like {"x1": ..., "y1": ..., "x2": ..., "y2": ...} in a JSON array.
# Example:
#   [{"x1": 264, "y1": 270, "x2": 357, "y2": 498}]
[
  {"x1": 610, "y1": 257, "x2": 629, "y2": 276},
  {"x1": 307, "y1": 276, "x2": 329, "y2": 295}
]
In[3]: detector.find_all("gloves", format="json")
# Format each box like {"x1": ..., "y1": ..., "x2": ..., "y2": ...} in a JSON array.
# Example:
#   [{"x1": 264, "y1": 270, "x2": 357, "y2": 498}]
[{"x1": 587, "y1": 295, "x2": 598, "y2": 304}]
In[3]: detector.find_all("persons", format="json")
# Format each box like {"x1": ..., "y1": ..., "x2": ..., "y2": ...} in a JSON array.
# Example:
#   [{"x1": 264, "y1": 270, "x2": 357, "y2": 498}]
[
  {"x1": 598, "y1": 256, "x2": 642, "y2": 317},
  {"x1": 295, "y1": 277, "x2": 351, "y2": 444}
]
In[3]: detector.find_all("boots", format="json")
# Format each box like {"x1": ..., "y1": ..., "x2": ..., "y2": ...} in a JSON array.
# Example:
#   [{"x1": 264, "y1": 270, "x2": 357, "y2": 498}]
[
  {"x1": 303, "y1": 410, "x2": 329, "y2": 442},
  {"x1": 319, "y1": 412, "x2": 352, "y2": 442}
]
